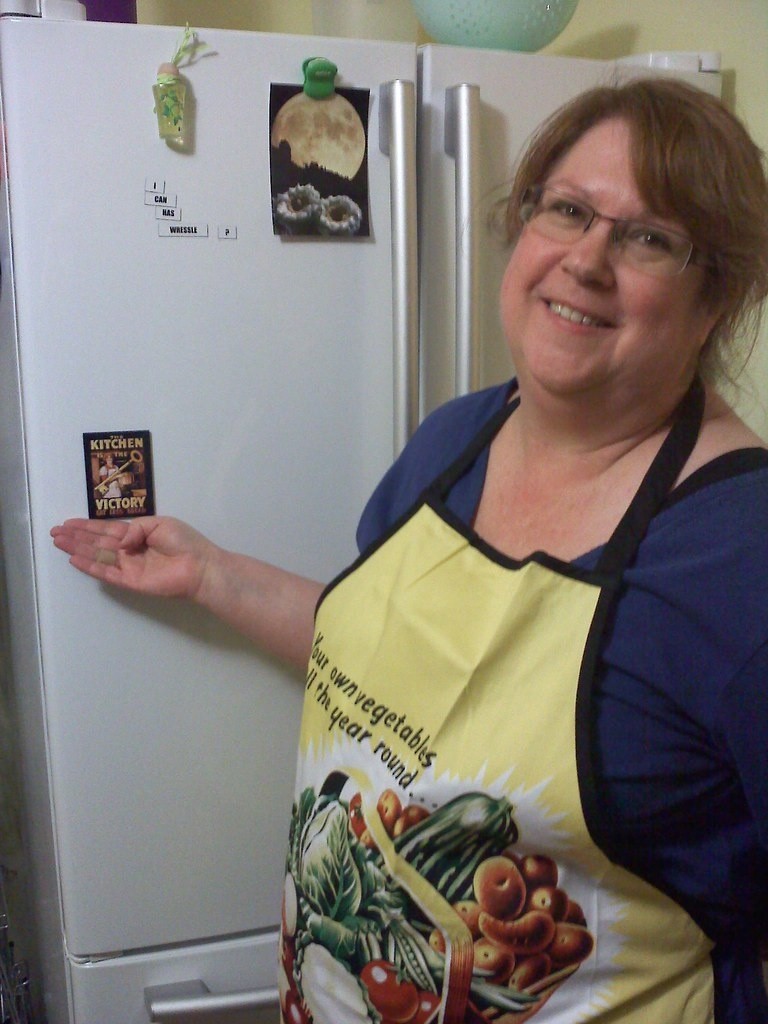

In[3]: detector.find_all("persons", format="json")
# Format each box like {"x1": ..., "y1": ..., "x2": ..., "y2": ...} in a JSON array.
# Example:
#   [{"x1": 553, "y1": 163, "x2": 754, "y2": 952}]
[{"x1": 50, "y1": 79, "x2": 768, "y2": 1024}]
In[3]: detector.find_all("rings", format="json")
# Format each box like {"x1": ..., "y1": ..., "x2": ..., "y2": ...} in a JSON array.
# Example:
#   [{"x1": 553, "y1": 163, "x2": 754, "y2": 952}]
[{"x1": 97, "y1": 548, "x2": 115, "y2": 564}]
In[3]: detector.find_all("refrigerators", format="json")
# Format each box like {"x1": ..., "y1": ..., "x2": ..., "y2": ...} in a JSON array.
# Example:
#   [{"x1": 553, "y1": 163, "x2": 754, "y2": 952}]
[{"x1": 0, "y1": 0, "x2": 723, "y2": 1024}]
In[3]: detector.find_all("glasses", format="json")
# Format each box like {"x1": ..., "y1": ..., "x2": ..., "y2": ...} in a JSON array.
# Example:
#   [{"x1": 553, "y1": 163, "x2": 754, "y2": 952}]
[{"x1": 517, "y1": 181, "x2": 719, "y2": 278}]
